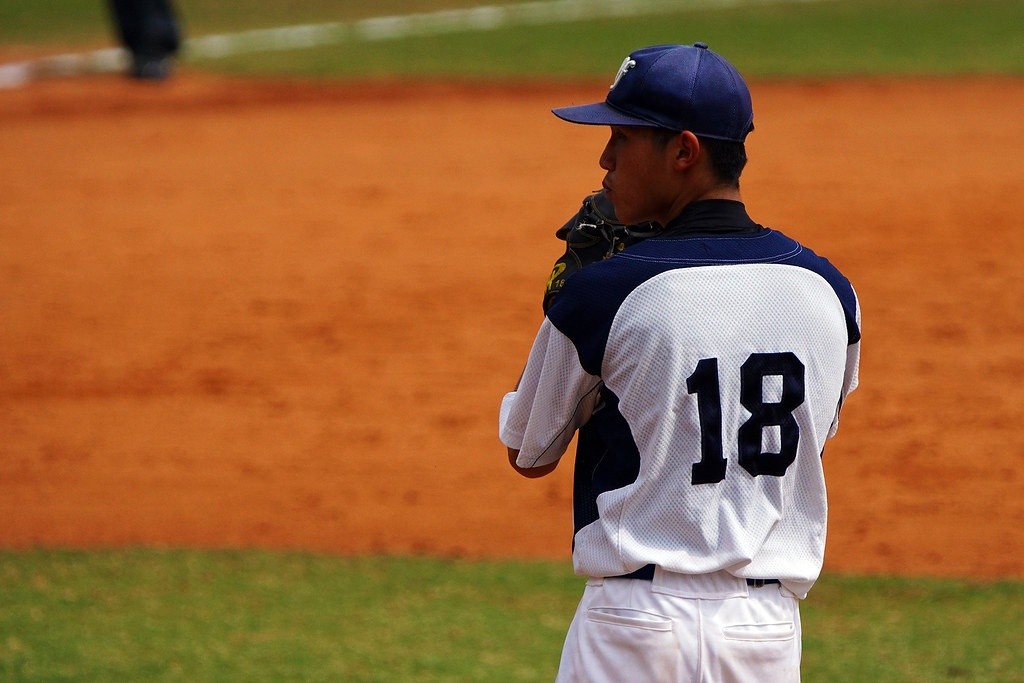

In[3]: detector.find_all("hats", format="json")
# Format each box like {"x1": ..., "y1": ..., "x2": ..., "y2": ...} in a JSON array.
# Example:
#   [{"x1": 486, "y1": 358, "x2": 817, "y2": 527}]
[{"x1": 549, "y1": 41, "x2": 755, "y2": 144}]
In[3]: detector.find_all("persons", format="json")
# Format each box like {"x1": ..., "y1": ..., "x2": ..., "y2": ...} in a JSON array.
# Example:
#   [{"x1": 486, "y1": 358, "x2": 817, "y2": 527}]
[
  {"x1": 499, "y1": 44, "x2": 861, "y2": 683},
  {"x1": 99, "y1": 0, "x2": 183, "y2": 78}
]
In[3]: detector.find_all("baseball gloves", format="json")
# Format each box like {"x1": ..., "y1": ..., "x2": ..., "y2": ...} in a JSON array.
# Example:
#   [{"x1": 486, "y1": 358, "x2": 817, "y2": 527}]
[{"x1": 543, "y1": 190, "x2": 664, "y2": 303}]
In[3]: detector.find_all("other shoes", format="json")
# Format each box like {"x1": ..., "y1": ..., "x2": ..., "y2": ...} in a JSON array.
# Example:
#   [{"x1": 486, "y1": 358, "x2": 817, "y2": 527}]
[{"x1": 131, "y1": 55, "x2": 171, "y2": 81}]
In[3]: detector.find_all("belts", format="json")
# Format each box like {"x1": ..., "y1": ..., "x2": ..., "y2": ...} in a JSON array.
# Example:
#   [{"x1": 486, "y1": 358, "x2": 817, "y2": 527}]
[{"x1": 605, "y1": 565, "x2": 782, "y2": 587}]
